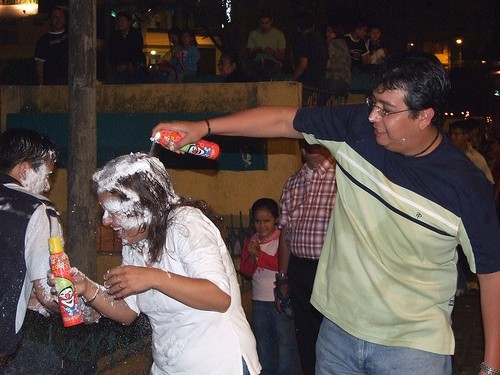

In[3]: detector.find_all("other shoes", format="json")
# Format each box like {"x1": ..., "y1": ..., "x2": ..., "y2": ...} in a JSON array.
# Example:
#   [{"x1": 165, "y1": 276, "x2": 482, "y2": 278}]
[
  {"x1": 468, "y1": 282, "x2": 479, "y2": 291},
  {"x1": 455, "y1": 289, "x2": 464, "y2": 297}
]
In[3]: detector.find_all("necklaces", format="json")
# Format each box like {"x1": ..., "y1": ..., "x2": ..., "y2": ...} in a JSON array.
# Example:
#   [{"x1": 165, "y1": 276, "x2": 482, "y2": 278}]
[{"x1": 408, "y1": 128, "x2": 440, "y2": 159}]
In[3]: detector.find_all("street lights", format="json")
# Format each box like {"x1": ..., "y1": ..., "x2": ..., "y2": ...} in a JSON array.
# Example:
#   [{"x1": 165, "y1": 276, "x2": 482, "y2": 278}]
[{"x1": 456, "y1": 39, "x2": 463, "y2": 62}]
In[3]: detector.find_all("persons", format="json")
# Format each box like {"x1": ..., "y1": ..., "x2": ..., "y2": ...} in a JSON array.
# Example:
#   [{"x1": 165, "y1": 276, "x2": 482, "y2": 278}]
[
  {"x1": 31, "y1": 6, "x2": 70, "y2": 85},
  {"x1": 447, "y1": 121, "x2": 500, "y2": 215},
  {"x1": 46, "y1": 152, "x2": 264, "y2": 375},
  {"x1": 238, "y1": 198, "x2": 305, "y2": 375},
  {"x1": 151, "y1": 51, "x2": 500, "y2": 375},
  {"x1": 97, "y1": 13, "x2": 151, "y2": 84},
  {"x1": 272, "y1": 138, "x2": 341, "y2": 375},
  {"x1": 159, "y1": 9, "x2": 395, "y2": 110},
  {"x1": 0, "y1": 126, "x2": 105, "y2": 375}
]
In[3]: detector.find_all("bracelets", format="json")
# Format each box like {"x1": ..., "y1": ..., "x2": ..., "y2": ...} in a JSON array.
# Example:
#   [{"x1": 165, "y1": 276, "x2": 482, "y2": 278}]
[
  {"x1": 204, "y1": 118, "x2": 211, "y2": 136},
  {"x1": 81, "y1": 283, "x2": 100, "y2": 305},
  {"x1": 277, "y1": 272, "x2": 288, "y2": 279},
  {"x1": 480, "y1": 362, "x2": 500, "y2": 375}
]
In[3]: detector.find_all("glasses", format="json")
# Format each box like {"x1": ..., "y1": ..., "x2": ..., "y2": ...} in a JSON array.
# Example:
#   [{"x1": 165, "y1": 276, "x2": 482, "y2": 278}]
[
  {"x1": 449, "y1": 131, "x2": 463, "y2": 137},
  {"x1": 366, "y1": 96, "x2": 412, "y2": 119}
]
